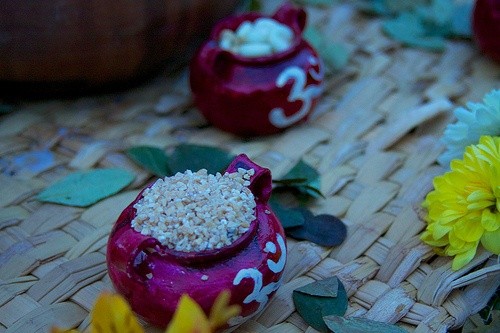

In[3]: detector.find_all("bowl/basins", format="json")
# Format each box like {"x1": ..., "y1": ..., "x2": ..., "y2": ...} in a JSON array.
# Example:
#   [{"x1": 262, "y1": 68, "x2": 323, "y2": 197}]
[
  {"x1": 189, "y1": 6, "x2": 324, "y2": 136},
  {"x1": 106, "y1": 153, "x2": 287, "y2": 333}
]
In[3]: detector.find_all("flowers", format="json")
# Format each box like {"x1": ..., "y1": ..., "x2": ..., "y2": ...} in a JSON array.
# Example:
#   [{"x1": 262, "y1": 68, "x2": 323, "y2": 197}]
[
  {"x1": 49, "y1": 288, "x2": 242, "y2": 333},
  {"x1": 419, "y1": 87, "x2": 500, "y2": 270}
]
